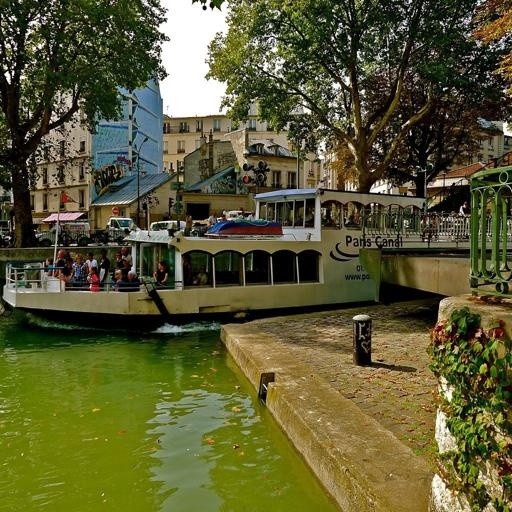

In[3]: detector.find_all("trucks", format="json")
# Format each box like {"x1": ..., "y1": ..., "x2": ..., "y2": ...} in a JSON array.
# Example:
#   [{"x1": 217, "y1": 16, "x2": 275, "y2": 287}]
[
  {"x1": 35, "y1": 222, "x2": 91, "y2": 246},
  {"x1": 89, "y1": 217, "x2": 135, "y2": 245},
  {"x1": 215, "y1": 210, "x2": 252, "y2": 222}
]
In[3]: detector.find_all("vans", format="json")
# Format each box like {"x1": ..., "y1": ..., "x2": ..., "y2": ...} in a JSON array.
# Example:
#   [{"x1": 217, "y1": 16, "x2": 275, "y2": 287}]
[{"x1": 149, "y1": 219, "x2": 187, "y2": 231}]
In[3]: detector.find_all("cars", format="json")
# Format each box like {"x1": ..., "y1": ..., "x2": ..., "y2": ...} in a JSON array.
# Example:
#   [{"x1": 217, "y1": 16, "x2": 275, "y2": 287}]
[{"x1": 0, "y1": 232, "x2": 12, "y2": 249}]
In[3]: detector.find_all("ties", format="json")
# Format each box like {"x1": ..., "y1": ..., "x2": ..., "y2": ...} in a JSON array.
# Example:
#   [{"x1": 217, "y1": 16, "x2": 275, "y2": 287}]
[{"x1": 117, "y1": 259, "x2": 119, "y2": 262}]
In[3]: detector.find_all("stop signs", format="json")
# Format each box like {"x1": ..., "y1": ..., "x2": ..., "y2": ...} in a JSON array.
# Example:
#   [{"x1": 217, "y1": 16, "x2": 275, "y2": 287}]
[{"x1": 111, "y1": 206, "x2": 120, "y2": 216}]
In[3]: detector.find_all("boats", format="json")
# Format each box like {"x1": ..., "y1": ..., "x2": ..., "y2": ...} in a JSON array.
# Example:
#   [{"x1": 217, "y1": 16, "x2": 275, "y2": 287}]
[{"x1": 1, "y1": 185, "x2": 456, "y2": 325}]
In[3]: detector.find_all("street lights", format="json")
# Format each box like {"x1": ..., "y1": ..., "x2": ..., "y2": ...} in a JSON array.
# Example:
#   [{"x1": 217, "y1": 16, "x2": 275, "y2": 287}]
[{"x1": 137, "y1": 137, "x2": 150, "y2": 226}]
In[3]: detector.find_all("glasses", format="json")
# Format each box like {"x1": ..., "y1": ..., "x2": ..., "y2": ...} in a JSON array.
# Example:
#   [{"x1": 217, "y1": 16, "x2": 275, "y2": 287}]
[{"x1": 116, "y1": 254, "x2": 120, "y2": 256}]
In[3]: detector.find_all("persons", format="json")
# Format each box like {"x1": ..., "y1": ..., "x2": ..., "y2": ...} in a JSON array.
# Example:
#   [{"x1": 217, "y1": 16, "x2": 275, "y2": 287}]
[
  {"x1": 44, "y1": 208, "x2": 254, "y2": 292},
  {"x1": 459, "y1": 201, "x2": 469, "y2": 219},
  {"x1": 300, "y1": 209, "x2": 312, "y2": 224}
]
